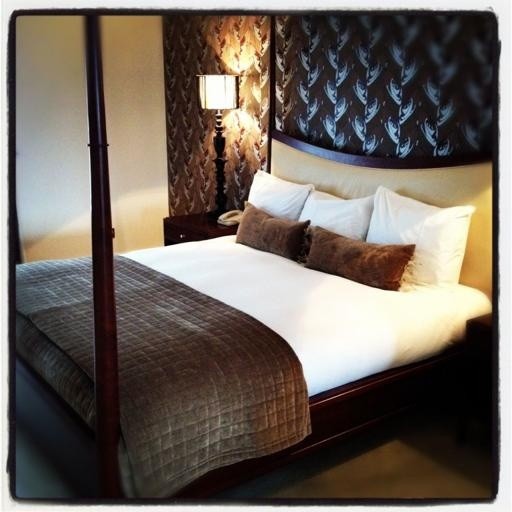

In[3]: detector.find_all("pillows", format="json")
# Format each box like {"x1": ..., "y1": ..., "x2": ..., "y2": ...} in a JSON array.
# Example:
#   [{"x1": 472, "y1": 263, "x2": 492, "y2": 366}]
[
  {"x1": 236, "y1": 200, "x2": 310, "y2": 263},
  {"x1": 248, "y1": 169, "x2": 315, "y2": 221},
  {"x1": 303, "y1": 225, "x2": 416, "y2": 290},
  {"x1": 365, "y1": 185, "x2": 476, "y2": 285},
  {"x1": 298, "y1": 189, "x2": 375, "y2": 241}
]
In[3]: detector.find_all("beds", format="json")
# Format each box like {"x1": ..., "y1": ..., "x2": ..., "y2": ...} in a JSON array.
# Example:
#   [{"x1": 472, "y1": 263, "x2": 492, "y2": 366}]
[{"x1": 16, "y1": 127, "x2": 496, "y2": 497}]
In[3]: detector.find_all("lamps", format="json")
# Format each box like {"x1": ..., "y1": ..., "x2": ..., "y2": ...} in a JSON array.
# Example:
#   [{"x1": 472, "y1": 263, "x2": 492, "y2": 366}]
[{"x1": 197, "y1": 73, "x2": 240, "y2": 211}]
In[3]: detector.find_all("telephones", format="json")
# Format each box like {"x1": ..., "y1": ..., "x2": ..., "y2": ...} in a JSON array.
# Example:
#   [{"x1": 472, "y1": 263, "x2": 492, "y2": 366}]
[{"x1": 217, "y1": 210, "x2": 242, "y2": 226}]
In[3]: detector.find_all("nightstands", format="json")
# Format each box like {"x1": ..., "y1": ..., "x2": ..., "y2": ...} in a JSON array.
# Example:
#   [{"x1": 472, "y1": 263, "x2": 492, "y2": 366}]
[{"x1": 164, "y1": 212, "x2": 238, "y2": 246}]
